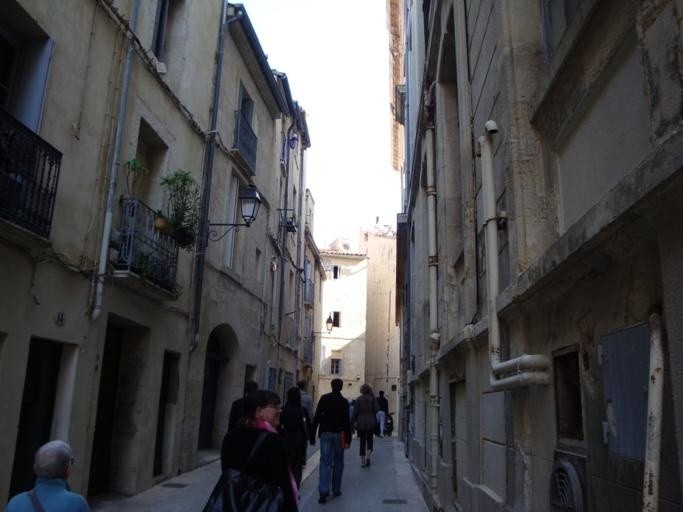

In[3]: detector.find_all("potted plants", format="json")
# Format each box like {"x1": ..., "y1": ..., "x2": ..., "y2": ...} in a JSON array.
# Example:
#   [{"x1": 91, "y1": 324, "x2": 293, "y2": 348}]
[
  {"x1": 159, "y1": 168, "x2": 201, "y2": 249},
  {"x1": 154, "y1": 208, "x2": 170, "y2": 232},
  {"x1": 124, "y1": 157, "x2": 149, "y2": 217}
]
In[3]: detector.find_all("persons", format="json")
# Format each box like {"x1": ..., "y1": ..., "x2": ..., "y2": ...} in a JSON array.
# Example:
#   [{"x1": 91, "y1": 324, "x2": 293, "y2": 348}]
[
  {"x1": 3, "y1": 440, "x2": 91, "y2": 512},
  {"x1": 202, "y1": 379, "x2": 388, "y2": 512}
]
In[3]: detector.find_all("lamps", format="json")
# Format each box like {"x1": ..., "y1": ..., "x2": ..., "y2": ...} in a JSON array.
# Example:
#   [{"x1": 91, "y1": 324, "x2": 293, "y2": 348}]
[{"x1": 205, "y1": 181, "x2": 263, "y2": 246}]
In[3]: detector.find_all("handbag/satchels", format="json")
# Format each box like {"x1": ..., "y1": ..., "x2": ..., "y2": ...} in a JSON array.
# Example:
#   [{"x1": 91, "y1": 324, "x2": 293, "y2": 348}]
[
  {"x1": 341, "y1": 431, "x2": 347, "y2": 449},
  {"x1": 202, "y1": 467, "x2": 299, "y2": 512}
]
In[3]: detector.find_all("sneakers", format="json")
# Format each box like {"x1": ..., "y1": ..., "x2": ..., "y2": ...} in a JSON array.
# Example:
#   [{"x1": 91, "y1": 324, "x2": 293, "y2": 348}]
[
  {"x1": 333, "y1": 492, "x2": 342, "y2": 496},
  {"x1": 362, "y1": 459, "x2": 370, "y2": 468},
  {"x1": 319, "y1": 493, "x2": 326, "y2": 505}
]
